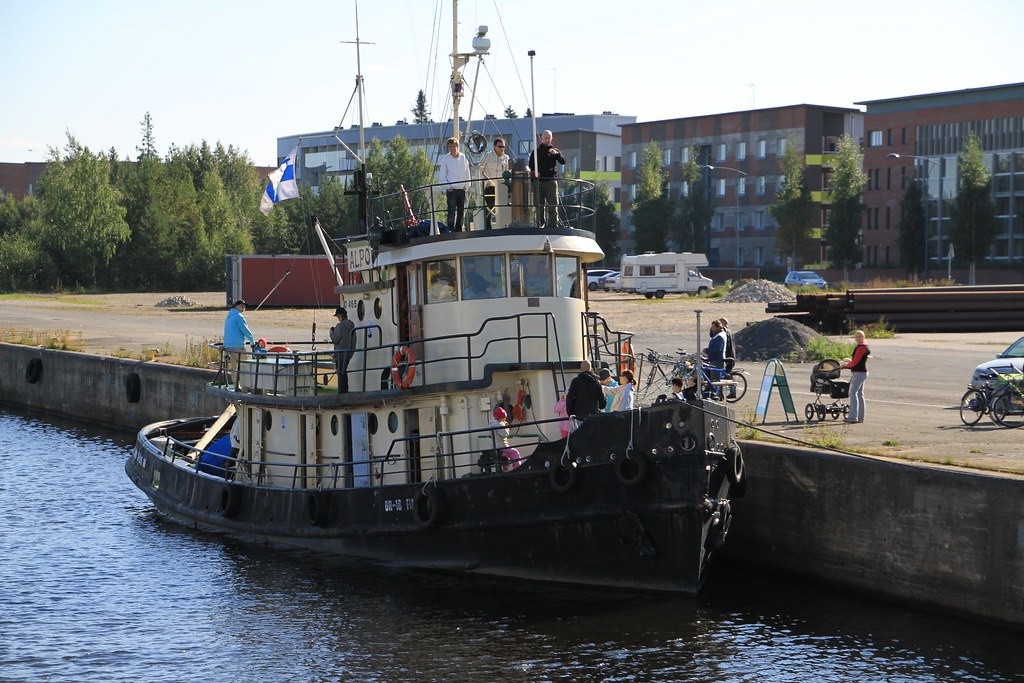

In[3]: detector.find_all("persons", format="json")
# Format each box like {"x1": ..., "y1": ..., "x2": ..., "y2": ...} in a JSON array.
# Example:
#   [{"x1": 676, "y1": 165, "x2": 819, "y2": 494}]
[
  {"x1": 223, "y1": 300, "x2": 255, "y2": 392},
  {"x1": 329, "y1": 306, "x2": 358, "y2": 394},
  {"x1": 668, "y1": 375, "x2": 698, "y2": 400},
  {"x1": 529, "y1": 130, "x2": 567, "y2": 228},
  {"x1": 553, "y1": 360, "x2": 637, "y2": 439},
  {"x1": 702, "y1": 318, "x2": 737, "y2": 398},
  {"x1": 525, "y1": 261, "x2": 549, "y2": 296},
  {"x1": 488, "y1": 407, "x2": 521, "y2": 472},
  {"x1": 439, "y1": 137, "x2": 472, "y2": 233},
  {"x1": 841, "y1": 330, "x2": 870, "y2": 423},
  {"x1": 478, "y1": 137, "x2": 510, "y2": 229}
]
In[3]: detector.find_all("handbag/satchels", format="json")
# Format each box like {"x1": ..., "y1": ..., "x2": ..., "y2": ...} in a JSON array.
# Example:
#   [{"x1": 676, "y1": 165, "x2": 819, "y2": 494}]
[{"x1": 830, "y1": 381, "x2": 849, "y2": 398}]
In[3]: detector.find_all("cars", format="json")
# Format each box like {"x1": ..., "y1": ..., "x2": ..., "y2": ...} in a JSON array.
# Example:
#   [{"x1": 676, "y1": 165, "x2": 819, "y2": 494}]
[
  {"x1": 971, "y1": 334, "x2": 1024, "y2": 398},
  {"x1": 598, "y1": 272, "x2": 634, "y2": 294}
]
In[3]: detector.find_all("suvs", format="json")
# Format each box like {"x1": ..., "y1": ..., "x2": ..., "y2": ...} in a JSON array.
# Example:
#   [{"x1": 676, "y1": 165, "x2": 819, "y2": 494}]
[
  {"x1": 586, "y1": 270, "x2": 614, "y2": 291},
  {"x1": 785, "y1": 272, "x2": 828, "y2": 289}
]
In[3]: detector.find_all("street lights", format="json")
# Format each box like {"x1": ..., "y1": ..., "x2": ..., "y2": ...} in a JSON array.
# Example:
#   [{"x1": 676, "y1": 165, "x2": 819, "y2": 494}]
[
  {"x1": 886, "y1": 153, "x2": 940, "y2": 278},
  {"x1": 703, "y1": 165, "x2": 748, "y2": 279}
]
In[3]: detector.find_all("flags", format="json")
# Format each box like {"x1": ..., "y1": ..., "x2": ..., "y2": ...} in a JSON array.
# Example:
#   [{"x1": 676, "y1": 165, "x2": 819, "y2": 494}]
[{"x1": 259, "y1": 141, "x2": 300, "y2": 216}]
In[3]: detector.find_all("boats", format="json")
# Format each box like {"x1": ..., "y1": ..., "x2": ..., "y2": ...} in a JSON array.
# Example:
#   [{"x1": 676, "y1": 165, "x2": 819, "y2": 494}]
[{"x1": 124, "y1": 0, "x2": 744, "y2": 593}]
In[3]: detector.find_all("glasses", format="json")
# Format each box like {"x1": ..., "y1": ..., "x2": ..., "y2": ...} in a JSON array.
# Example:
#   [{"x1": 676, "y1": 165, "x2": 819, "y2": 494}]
[{"x1": 498, "y1": 146, "x2": 506, "y2": 148}]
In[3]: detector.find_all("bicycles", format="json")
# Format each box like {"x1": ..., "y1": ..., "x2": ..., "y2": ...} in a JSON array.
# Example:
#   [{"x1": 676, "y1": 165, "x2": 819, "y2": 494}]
[
  {"x1": 960, "y1": 373, "x2": 1024, "y2": 428},
  {"x1": 643, "y1": 347, "x2": 748, "y2": 403}
]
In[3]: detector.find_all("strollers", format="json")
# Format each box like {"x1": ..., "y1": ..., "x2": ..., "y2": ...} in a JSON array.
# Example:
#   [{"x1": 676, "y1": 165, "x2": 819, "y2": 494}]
[{"x1": 804, "y1": 357, "x2": 851, "y2": 420}]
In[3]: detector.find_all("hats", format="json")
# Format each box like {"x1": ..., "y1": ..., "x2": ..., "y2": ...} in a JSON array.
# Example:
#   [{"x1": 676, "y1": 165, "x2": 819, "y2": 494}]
[
  {"x1": 494, "y1": 407, "x2": 507, "y2": 419},
  {"x1": 334, "y1": 307, "x2": 347, "y2": 316},
  {"x1": 598, "y1": 368, "x2": 610, "y2": 380}
]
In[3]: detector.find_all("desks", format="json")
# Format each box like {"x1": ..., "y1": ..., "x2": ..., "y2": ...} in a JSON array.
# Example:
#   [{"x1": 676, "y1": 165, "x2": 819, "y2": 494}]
[{"x1": 237, "y1": 358, "x2": 314, "y2": 396}]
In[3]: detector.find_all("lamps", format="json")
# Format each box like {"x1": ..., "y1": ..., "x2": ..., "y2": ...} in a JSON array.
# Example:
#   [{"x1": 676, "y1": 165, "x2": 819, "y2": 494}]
[
  {"x1": 495, "y1": 390, "x2": 504, "y2": 401},
  {"x1": 543, "y1": 235, "x2": 554, "y2": 254}
]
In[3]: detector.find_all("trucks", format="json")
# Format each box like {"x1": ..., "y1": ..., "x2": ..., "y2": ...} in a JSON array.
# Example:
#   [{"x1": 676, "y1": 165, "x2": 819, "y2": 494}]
[{"x1": 620, "y1": 252, "x2": 714, "y2": 299}]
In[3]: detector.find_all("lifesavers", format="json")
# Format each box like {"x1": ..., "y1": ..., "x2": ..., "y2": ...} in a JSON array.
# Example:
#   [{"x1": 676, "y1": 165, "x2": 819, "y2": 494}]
[
  {"x1": 26, "y1": 358, "x2": 42, "y2": 384},
  {"x1": 125, "y1": 372, "x2": 141, "y2": 403},
  {"x1": 304, "y1": 492, "x2": 329, "y2": 527},
  {"x1": 714, "y1": 503, "x2": 732, "y2": 546},
  {"x1": 412, "y1": 486, "x2": 448, "y2": 529},
  {"x1": 614, "y1": 452, "x2": 648, "y2": 487},
  {"x1": 391, "y1": 345, "x2": 416, "y2": 389},
  {"x1": 621, "y1": 341, "x2": 635, "y2": 374},
  {"x1": 725, "y1": 446, "x2": 743, "y2": 485},
  {"x1": 217, "y1": 484, "x2": 241, "y2": 518},
  {"x1": 548, "y1": 458, "x2": 580, "y2": 495}
]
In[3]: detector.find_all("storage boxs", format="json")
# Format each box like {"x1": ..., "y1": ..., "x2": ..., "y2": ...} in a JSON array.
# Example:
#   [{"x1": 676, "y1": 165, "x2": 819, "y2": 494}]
[{"x1": 225, "y1": 253, "x2": 362, "y2": 306}]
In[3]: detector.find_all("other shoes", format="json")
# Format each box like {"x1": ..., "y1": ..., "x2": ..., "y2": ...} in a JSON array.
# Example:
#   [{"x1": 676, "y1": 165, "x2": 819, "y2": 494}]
[
  {"x1": 726, "y1": 390, "x2": 737, "y2": 399},
  {"x1": 843, "y1": 418, "x2": 859, "y2": 423}
]
